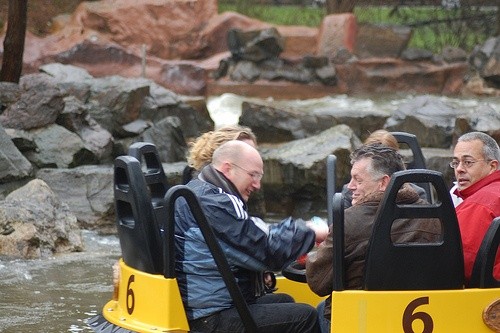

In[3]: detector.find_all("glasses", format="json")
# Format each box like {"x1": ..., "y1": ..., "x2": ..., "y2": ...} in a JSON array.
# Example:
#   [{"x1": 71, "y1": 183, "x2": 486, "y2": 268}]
[
  {"x1": 449, "y1": 158, "x2": 492, "y2": 167},
  {"x1": 230, "y1": 161, "x2": 264, "y2": 180}
]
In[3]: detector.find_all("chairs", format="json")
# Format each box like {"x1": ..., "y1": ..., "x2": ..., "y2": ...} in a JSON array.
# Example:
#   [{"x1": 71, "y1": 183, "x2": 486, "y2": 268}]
[{"x1": 114, "y1": 132, "x2": 500, "y2": 291}]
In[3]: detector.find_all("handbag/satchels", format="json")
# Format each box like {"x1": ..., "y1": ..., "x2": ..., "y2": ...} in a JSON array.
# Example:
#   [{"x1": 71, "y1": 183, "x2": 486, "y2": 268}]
[{"x1": 247, "y1": 270, "x2": 266, "y2": 296}]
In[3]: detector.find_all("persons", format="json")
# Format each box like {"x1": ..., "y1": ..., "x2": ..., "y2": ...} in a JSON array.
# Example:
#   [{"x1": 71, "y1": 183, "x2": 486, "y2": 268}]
[
  {"x1": 338, "y1": 130, "x2": 430, "y2": 202},
  {"x1": 168, "y1": 140, "x2": 324, "y2": 333},
  {"x1": 184, "y1": 125, "x2": 271, "y2": 223},
  {"x1": 440, "y1": 132, "x2": 500, "y2": 285},
  {"x1": 306, "y1": 142, "x2": 460, "y2": 333}
]
